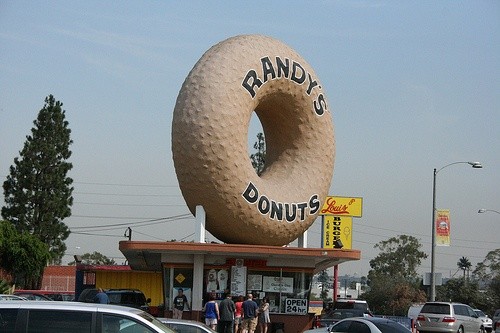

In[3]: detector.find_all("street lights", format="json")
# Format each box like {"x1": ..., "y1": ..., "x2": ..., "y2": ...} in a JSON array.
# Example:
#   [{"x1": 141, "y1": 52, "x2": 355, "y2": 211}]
[
  {"x1": 345, "y1": 272, "x2": 358, "y2": 298},
  {"x1": 430, "y1": 160, "x2": 483, "y2": 302}
]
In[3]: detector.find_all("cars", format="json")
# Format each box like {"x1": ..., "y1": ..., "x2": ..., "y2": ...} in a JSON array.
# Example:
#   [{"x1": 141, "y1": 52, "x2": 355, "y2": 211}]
[
  {"x1": 407, "y1": 302, "x2": 500, "y2": 333},
  {"x1": 0, "y1": 287, "x2": 220, "y2": 333},
  {"x1": 302, "y1": 315, "x2": 414, "y2": 333},
  {"x1": 306, "y1": 295, "x2": 376, "y2": 329}
]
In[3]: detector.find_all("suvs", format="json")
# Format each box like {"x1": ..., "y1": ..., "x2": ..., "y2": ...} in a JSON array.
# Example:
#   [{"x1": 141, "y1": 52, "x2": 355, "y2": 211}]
[
  {"x1": 0, "y1": 299, "x2": 177, "y2": 333},
  {"x1": 414, "y1": 301, "x2": 485, "y2": 333}
]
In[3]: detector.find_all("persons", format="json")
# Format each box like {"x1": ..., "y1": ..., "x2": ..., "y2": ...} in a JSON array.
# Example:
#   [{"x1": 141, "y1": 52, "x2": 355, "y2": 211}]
[
  {"x1": 261, "y1": 297, "x2": 270, "y2": 333},
  {"x1": 204, "y1": 294, "x2": 220, "y2": 331},
  {"x1": 220, "y1": 294, "x2": 236, "y2": 333},
  {"x1": 234, "y1": 295, "x2": 244, "y2": 333},
  {"x1": 241, "y1": 294, "x2": 258, "y2": 333},
  {"x1": 172, "y1": 288, "x2": 190, "y2": 319},
  {"x1": 94, "y1": 288, "x2": 110, "y2": 305}
]
